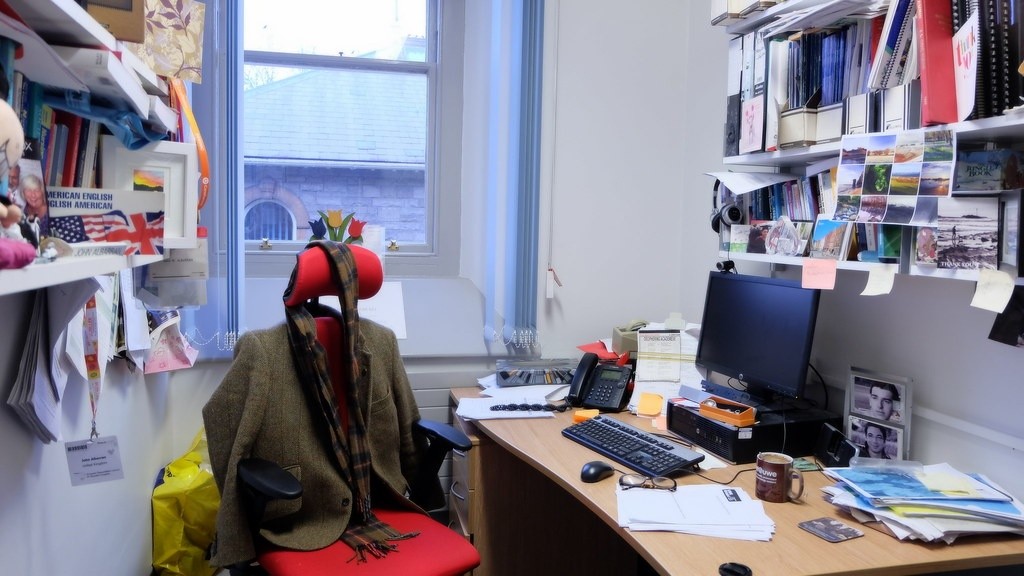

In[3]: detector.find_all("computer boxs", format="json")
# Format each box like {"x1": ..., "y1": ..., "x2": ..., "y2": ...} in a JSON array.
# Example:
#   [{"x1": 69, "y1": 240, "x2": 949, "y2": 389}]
[{"x1": 667, "y1": 397, "x2": 842, "y2": 466}]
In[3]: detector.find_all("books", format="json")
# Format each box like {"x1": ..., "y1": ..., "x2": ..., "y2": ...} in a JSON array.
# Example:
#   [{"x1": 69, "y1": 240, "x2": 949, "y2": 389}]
[
  {"x1": 0, "y1": 0, "x2": 179, "y2": 189},
  {"x1": 719, "y1": 0, "x2": 1024, "y2": 277}
]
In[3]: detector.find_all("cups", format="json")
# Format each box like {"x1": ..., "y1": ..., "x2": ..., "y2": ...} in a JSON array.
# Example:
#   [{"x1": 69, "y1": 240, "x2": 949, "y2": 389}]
[{"x1": 755, "y1": 452, "x2": 804, "y2": 503}]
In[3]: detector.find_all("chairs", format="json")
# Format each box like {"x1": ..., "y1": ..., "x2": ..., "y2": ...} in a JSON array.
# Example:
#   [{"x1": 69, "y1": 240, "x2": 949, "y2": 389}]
[{"x1": 239, "y1": 244, "x2": 482, "y2": 576}]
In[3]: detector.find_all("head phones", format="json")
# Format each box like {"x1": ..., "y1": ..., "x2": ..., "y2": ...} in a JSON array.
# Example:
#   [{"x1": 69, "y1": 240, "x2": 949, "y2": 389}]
[{"x1": 711, "y1": 179, "x2": 743, "y2": 233}]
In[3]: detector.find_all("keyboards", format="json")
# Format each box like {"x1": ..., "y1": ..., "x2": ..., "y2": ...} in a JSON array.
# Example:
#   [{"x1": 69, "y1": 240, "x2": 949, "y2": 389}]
[{"x1": 561, "y1": 415, "x2": 705, "y2": 479}]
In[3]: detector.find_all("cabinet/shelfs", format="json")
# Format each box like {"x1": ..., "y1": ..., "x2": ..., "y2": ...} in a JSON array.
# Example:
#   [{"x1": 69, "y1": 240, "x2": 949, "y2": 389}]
[
  {"x1": 0, "y1": 0, "x2": 171, "y2": 294},
  {"x1": 723, "y1": 0, "x2": 1024, "y2": 287}
]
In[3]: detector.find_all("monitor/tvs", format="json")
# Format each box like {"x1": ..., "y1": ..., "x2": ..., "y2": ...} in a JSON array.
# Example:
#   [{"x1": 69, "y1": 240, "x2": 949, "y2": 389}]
[{"x1": 694, "y1": 270, "x2": 821, "y2": 419}]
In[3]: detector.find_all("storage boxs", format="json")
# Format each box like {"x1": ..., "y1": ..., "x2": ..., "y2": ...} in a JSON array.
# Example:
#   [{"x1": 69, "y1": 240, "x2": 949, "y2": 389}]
[
  {"x1": 710, "y1": 1, "x2": 781, "y2": 28},
  {"x1": 773, "y1": 80, "x2": 922, "y2": 149}
]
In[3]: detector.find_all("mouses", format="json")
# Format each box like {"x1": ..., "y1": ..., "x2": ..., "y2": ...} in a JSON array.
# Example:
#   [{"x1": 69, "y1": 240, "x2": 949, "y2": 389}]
[{"x1": 580, "y1": 460, "x2": 615, "y2": 483}]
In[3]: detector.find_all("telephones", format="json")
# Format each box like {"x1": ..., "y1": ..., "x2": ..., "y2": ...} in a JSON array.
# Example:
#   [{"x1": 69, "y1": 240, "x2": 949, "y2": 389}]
[{"x1": 566, "y1": 352, "x2": 633, "y2": 415}]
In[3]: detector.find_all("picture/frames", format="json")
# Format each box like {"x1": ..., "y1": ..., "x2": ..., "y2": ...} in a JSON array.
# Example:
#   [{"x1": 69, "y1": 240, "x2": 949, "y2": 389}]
[
  {"x1": 84, "y1": 0, "x2": 145, "y2": 44},
  {"x1": 99, "y1": 134, "x2": 199, "y2": 248}
]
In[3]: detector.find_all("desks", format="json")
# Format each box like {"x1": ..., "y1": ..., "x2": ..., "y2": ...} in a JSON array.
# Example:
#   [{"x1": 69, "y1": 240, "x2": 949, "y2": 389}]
[{"x1": 449, "y1": 387, "x2": 1024, "y2": 576}]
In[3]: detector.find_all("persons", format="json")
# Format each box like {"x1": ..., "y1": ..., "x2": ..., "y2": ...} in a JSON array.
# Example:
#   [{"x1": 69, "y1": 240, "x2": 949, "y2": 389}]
[
  {"x1": 870, "y1": 381, "x2": 900, "y2": 421},
  {"x1": 756, "y1": 226, "x2": 771, "y2": 243},
  {"x1": 750, "y1": 224, "x2": 760, "y2": 241},
  {"x1": 6, "y1": 164, "x2": 49, "y2": 238},
  {"x1": 855, "y1": 423, "x2": 890, "y2": 460}
]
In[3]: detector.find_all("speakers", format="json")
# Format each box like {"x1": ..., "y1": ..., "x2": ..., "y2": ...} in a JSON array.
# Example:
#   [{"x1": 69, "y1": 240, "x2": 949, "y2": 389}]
[{"x1": 811, "y1": 422, "x2": 869, "y2": 468}]
